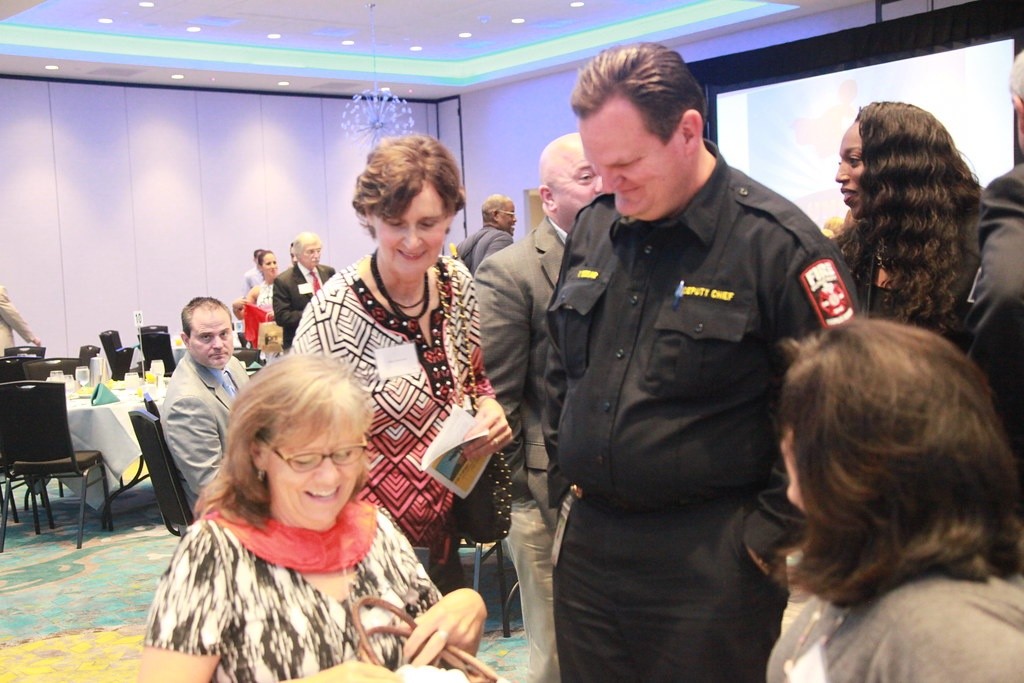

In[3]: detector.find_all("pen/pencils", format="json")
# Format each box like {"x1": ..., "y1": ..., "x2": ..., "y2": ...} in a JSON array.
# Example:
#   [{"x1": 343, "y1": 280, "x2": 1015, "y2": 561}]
[{"x1": 672, "y1": 280, "x2": 684, "y2": 311}]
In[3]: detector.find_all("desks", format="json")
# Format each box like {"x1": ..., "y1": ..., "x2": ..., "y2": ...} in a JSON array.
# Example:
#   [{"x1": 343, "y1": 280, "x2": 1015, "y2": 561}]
[
  {"x1": 66, "y1": 377, "x2": 173, "y2": 532},
  {"x1": 168, "y1": 338, "x2": 188, "y2": 368}
]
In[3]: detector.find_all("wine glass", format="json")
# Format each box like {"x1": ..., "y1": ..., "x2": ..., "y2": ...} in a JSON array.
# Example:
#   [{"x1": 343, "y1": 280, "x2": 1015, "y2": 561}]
[{"x1": 150, "y1": 359, "x2": 165, "y2": 394}]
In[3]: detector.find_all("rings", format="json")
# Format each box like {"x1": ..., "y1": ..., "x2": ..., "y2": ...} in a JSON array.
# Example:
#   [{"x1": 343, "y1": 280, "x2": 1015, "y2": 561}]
[{"x1": 494, "y1": 438, "x2": 499, "y2": 446}]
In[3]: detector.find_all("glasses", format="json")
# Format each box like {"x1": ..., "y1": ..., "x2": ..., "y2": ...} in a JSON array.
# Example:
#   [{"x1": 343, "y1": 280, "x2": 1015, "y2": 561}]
[
  {"x1": 491, "y1": 209, "x2": 515, "y2": 218},
  {"x1": 254, "y1": 432, "x2": 369, "y2": 473}
]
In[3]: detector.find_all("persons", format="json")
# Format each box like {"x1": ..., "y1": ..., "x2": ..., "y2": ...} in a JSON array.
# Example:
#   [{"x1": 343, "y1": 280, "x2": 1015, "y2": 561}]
[
  {"x1": 161, "y1": 296, "x2": 250, "y2": 513},
  {"x1": 135, "y1": 355, "x2": 485, "y2": 683},
  {"x1": 273, "y1": 233, "x2": 335, "y2": 357},
  {"x1": 288, "y1": 135, "x2": 515, "y2": 593},
  {"x1": 542, "y1": 43, "x2": 857, "y2": 683},
  {"x1": 453, "y1": 194, "x2": 517, "y2": 278},
  {"x1": 475, "y1": 133, "x2": 605, "y2": 530},
  {"x1": 966, "y1": 52, "x2": 1024, "y2": 460},
  {"x1": 0, "y1": 286, "x2": 41, "y2": 357},
  {"x1": 833, "y1": 103, "x2": 979, "y2": 340},
  {"x1": 765, "y1": 320, "x2": 1024, "y2": 683},
  {"x1": 233, "y1": 243, "x2": 298, "y2": 321}
]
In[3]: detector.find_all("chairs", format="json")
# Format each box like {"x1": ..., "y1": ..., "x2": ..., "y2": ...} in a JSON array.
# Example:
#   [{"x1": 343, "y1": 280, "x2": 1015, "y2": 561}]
[
  {"x1": 0, "y1": 355, "x2": 41, "y2": 383},
  {"x1": 0, "y1": 380, "x2": 113, "y2": 552},
  {"x1": 127, "y1": 410, "x2": 196, "y2": 537},
  {"x1": 448, "y1": 474, "x2": 520, "y2": 638},
  {"x1": 232, "y1": 348, "x2": 261, "y2": 371},
  {"x1": 99, "y1": 330, "x2": 134, "y2": 380},
  {"x1": 137, "y1": 331, "x2": 176, "y2": 376},
  {"x1": 138, "y1": 325, "x2": 169, "y2": 333},
  {"x1": 79, "y1": 345, "x2": 100, "y2": 367},
  {"x1": 22, "y1": 358, "x2": 83, "y2": 382},
  {"x1": 144, "y1": 392, "x2": 161, "y2": 418},
  {"x1": 3, "y1": 345, "x2": 46, "y2": 359},
  {"x1": 238, "y1": 331, "x2": 252, "y2": 348}
]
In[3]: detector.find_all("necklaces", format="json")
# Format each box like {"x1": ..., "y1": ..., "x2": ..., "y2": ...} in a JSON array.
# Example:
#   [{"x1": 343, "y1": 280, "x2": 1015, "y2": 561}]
[{"x1": 371, "y1": 249, "x2": 430, "y2": 320}]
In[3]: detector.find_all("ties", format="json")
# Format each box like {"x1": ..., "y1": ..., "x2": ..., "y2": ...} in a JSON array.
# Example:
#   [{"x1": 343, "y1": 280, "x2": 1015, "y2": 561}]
[{"x1": 310, "y1": 271, "x2": 321, "y2": 294}]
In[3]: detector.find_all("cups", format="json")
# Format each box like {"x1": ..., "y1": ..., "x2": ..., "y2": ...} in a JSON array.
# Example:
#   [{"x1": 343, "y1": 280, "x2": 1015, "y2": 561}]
[
  {"x1": 50, "y1": 370, "x2": 63, "y2": 382},
  {"x1": 75, "y1": 366, "x2": 90, "y2": 386},
  {"x1": 125, "y1": 372, "x2": 139, "y2": 395},
  {"x1": 63, "y1": 375, "x2": 74, "y2": 397}
]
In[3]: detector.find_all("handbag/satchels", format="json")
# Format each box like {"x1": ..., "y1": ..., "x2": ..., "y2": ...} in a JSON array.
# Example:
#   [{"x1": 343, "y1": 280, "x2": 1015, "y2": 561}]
[
  {"x1": 451, "y1": 448, "x2": 513, "y2": 544},
  {"x1": 350, "y1": 595, "x2": 513, "y2": 683}
]
